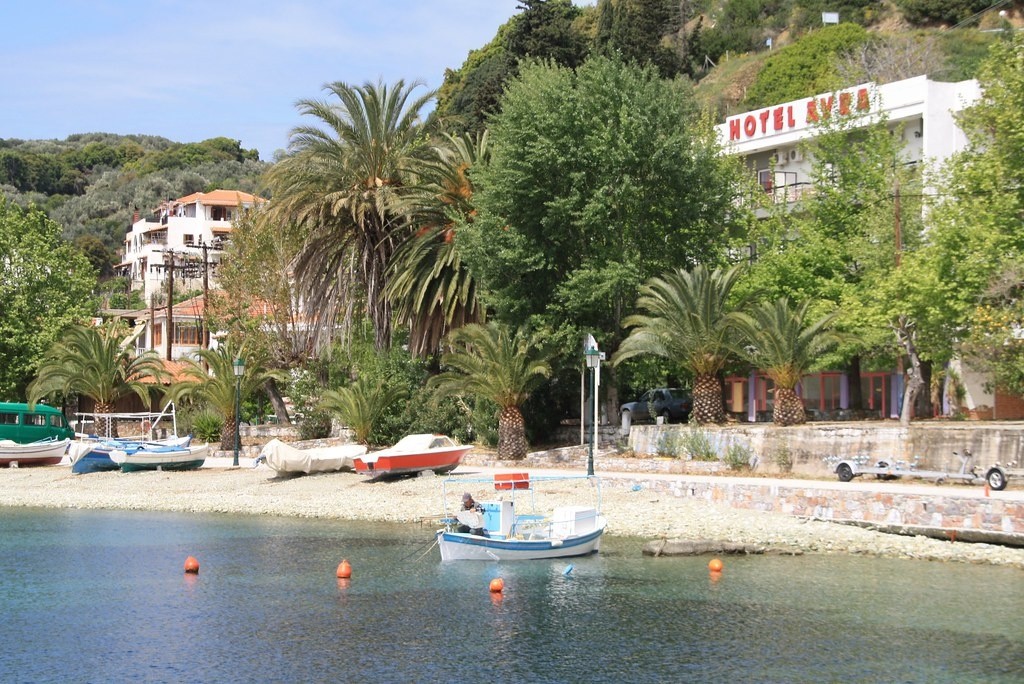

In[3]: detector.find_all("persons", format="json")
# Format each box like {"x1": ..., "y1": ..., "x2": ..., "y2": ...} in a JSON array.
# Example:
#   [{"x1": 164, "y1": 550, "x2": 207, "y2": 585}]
[{"x1": 452, "y1": 491, "x2": 486, "y2": 537}]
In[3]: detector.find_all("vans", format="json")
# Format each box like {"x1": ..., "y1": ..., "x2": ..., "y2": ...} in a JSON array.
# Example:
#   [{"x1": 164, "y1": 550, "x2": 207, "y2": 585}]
[{"x1": 0, "y1": 402, "x2": 75, "y2": 444}]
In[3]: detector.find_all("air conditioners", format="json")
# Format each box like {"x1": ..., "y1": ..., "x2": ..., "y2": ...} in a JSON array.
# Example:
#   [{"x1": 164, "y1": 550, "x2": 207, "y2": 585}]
[
  {"x1": 773, "y1": 152, "x2": 786, "y2": 164},
  {"x1": 788, "y1": 147, "x2": 802, "y2": 162}
]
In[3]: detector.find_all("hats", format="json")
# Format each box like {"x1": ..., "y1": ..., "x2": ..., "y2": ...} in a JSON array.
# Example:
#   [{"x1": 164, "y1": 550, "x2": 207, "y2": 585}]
[{"x1": 461, "y1": 493, "x2": 471, "y2": 502}]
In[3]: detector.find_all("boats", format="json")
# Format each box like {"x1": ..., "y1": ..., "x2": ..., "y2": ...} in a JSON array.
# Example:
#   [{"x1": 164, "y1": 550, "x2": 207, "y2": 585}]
[
  {"x1": 110, "y1": 443, "x2": 211, "y2": 471},
  {"x1": 262, "y1": 437, "x2": 370, "y2": 474},
  {"x1": 354, "y1": 435, "x2": 475, "y2": 477},
  {"x1": 435, "y1": 472, "x2": 608, "y2": 562},
  {"x1": 0, "y1": 435, "x2": 69, "y2": 466},
  {"x1": 70, "y1": 400, "x2": 197, "y2": 474}
]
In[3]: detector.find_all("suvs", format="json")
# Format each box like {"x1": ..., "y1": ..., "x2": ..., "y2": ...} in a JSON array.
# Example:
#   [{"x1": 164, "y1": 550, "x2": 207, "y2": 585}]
[{"x1": 618, "y1": 385, "x2": 693, "y2": 425}]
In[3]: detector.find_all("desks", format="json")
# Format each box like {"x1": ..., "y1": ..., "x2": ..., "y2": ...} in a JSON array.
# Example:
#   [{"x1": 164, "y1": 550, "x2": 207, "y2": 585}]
[{"x1": 511, "y1": 515, "x2": 551, "y2": 538}]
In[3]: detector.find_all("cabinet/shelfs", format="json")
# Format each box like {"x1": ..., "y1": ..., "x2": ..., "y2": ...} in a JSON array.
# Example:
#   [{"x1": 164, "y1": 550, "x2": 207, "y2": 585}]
[{"x1": 478, "y1": 500, "x2": 514, "y2": 536}]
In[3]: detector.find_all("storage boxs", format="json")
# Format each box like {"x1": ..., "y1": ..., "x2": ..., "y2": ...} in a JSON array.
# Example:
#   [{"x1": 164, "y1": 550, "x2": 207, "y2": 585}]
[{"x1": 494, "y1": 473, "x2": 529, "y2": 490}]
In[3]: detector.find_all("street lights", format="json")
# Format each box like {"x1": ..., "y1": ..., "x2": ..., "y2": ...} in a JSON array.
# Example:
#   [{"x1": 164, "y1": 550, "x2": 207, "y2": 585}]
[
  {"x1": 233, "y1": 356, "x2": 247, "y2": 466},
  {"x1": 585, "y1": 346, "x2": 600, "y2": 477}
]
